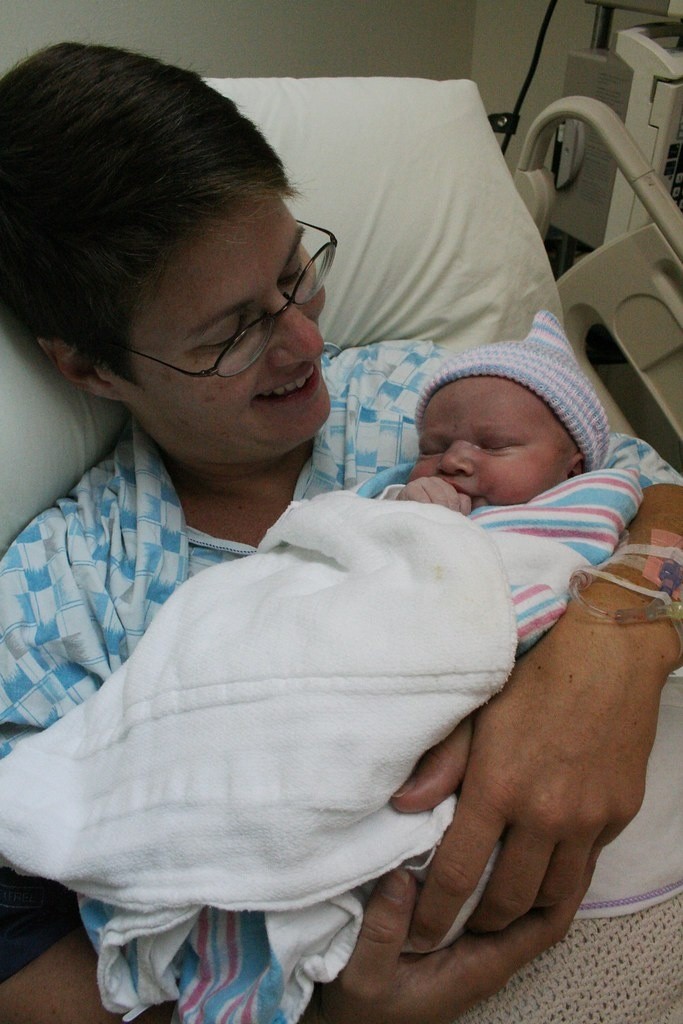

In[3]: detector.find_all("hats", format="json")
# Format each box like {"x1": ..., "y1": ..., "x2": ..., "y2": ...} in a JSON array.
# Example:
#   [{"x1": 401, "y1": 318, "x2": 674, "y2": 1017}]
[{"x1": 414, "y1": 309, "x2": 611, "y2": 471}]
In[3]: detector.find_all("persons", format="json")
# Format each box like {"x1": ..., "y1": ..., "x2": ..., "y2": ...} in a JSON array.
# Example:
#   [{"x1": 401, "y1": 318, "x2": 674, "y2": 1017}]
[
  {"x1": 1, "y1": 311, "x2": 648, "y2": 1023},
  {"x1": 0, "y1": 43, "x2": 683, "y2": 1024}
]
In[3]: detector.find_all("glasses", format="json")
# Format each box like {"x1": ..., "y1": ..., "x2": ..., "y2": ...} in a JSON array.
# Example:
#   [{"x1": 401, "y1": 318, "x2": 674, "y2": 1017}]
[{"x1": 121, "y1": 217, "x2": 336, "y2": 378}]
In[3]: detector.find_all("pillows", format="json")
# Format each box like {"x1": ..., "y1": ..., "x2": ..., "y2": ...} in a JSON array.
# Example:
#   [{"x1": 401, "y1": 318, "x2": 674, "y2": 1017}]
[{"x1": 0, "y1": 76, "x2": 564, "y2": 561}]
